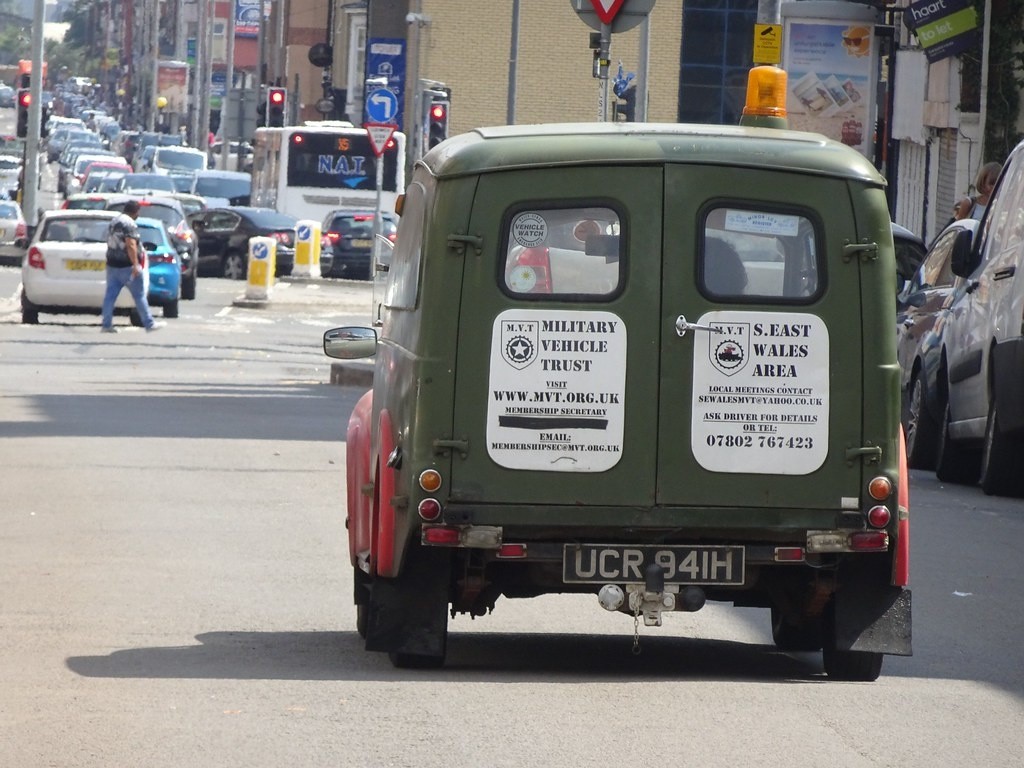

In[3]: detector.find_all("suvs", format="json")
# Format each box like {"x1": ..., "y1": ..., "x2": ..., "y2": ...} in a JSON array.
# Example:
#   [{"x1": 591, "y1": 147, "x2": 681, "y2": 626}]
[{"x1": 103, "y1": 194, "x2": 206, "y2": 301}]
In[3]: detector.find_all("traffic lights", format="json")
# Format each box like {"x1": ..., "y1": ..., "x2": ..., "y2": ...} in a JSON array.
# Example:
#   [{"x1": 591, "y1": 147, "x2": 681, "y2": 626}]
[
  {"x1": 265, "y1": 86, "x2": 288, "y2": 129},
  {"x1": 256, "y1": 100, "x2": 267, "y2": 127},
  {"x1": 429, "y1": 100, "x2": 449, "y2": 151},
  {"x1": 16, "y1": 88, "x2": 29, "y2": 140},
  {"x1": 617, "y1": 83, "x2": 637, "y2": 122}
]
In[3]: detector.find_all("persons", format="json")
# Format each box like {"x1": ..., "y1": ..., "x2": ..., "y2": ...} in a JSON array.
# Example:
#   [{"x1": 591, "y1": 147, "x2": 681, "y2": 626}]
[
  {"x1": 703, "y1": 237, "x2": 748, "y2": 295},
  {"x1": 100, "y1": 200, "x2": 168, "y2": 335},
  {"x1": 123, "y1": 140, "x2": 133, "y2": 164},
  {"x1": 956, "y1": 162, "x2": 1003, "y2": 222},
  {"x1": 87, "y1": 112, "x2": 96, "y2": 132}
]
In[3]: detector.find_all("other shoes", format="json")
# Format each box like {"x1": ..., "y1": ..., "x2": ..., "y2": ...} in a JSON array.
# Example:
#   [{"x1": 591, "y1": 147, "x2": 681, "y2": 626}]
[
  {"x1": 146, "y1": 321, "x2": 166, "y2": 332},
  {"x1": 101, "y1": 327, "x2": 117, "y2": 333}
]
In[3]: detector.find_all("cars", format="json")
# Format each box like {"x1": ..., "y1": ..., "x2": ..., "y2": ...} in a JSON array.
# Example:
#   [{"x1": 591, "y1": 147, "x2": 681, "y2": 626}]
[
  {"x1": 0, "y1": 199, "x2": 28, "y2": 265},
  {"x1": 320, "y1": 210, "x2": 400, "y2": 280},
  {"x1": 0, "y1": 75, "x2": 255, "y2": 228},
  {"x1": 14, "y1": 209, "x2": 158, "y2": 328},
  {"x1": 791, "y1": 210, "x2": 932, "y2": 309},
  {"x1": 933, "y1": 142, "x2": 1024, "y2": 496},
  {"x1": 187, "y1": 205, "x2": 335, "y2": 280},
  {"x1": 899, "y1": 217, "x2": 982, "y2": 470},
  {"x1": 75, "y1": 216, "x2": 181, "y2": 320}
]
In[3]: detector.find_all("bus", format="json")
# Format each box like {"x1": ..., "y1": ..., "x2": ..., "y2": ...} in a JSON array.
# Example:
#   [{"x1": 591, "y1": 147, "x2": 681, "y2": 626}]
[{"x1": 249, "y1": 120, "x2": 406, "y2": 228}]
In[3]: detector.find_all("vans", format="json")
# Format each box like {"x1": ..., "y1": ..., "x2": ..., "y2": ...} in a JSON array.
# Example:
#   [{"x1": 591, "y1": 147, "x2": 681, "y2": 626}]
[{"x1": 324, "y1": 66, "x2": 914, "y2": 682}]
